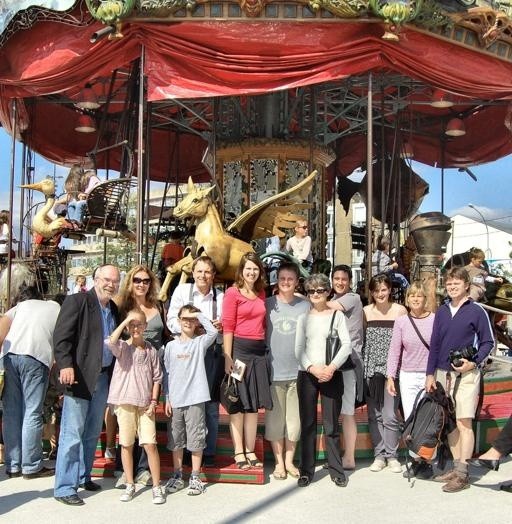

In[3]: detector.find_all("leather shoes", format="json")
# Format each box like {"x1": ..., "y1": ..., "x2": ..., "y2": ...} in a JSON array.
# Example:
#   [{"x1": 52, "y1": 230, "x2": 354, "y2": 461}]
[
  {"x1": 9, "y1": 465, "x2": 54, "y2": 480},
  {"x1": 79, "y1": 481, "x2": 101, "y2": 491},
  {"x1": 466, "y1": 457, "x2": 500, "y2": 471},
  {"x1": 55, "y1": 494, "x2": 83, "y2": 505}
]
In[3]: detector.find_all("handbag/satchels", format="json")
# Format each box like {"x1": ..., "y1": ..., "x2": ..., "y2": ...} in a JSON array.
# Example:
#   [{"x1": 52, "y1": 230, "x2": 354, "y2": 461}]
[
  {"x1": 325, "y1": 311, "x2": 355, "y2": 370},
  {"x1": 220, "y1": 374, "x2": 243, "y2": 414}
]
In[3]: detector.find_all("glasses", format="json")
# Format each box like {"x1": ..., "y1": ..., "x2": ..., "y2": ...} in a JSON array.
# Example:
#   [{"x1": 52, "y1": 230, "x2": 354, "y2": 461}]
[
  {"x1": 298, "y1": 226, "x2": 307, "y2": 229},
  {"x1": 308, "y1": 289, "x2": 327, "y2": 294},
  {"x1": 133, "y1": 277, "x2": 150, "y2": 285}
]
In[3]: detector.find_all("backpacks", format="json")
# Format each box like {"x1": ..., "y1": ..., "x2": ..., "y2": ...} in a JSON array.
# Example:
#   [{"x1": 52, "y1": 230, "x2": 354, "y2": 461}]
[{"x1": 399, "y1": 387, "x2": 448, "y2": 464}]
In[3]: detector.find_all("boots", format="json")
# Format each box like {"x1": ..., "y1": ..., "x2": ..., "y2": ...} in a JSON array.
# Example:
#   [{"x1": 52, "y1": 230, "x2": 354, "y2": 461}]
[{"x1": 433, "y1": 459, "x2": 470, "y2": 493}]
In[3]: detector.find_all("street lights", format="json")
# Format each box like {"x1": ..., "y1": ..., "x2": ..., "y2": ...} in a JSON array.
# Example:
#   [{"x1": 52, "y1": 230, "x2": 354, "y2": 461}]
[{"x1": 466, "y1": 202, "x2": 491, "y2": 259}]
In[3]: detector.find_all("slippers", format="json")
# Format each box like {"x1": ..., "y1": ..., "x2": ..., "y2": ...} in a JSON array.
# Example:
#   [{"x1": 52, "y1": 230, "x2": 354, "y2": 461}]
[
  {"x1": 288, "y1": 470, "x2": 300, "y2": 477},
  {"x1": 272, "y1": 471, "x2": 286, "y2": 480}
]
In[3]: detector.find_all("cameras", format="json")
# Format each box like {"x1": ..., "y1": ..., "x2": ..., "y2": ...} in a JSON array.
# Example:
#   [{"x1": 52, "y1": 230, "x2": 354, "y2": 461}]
[{"x1": 448, "y1": 345, "x2": 477, "y2": 369}]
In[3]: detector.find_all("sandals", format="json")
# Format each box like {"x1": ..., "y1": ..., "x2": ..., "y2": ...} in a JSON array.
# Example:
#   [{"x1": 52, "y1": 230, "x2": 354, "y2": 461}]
[
  {"x1": 106, "y1": 449, "x2": 116, "y2": 461},
  {"x1": 233, "y1": 451, "x2": 263, "y2": 469}
]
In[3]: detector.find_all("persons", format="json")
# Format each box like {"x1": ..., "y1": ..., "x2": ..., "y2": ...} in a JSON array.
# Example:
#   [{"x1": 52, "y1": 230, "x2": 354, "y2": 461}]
[{"x1": 0, "y1": 165, "x2": 512, "y2": 506}]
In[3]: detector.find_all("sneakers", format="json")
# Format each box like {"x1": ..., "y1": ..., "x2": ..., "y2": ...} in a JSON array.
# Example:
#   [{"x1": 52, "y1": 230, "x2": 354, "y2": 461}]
[
  {"x1": 114, "y1": 455, "x2": 216, "y2": 504},
  {"x1": 333, "y1": 477, "x2": 347, "y2": 487},
  {"x1": 369, "y1": 459, "x2": 432, "y2": 479},
  {"x1": 297, "y1": 476, "x2": 311, "y2": 487}
]
